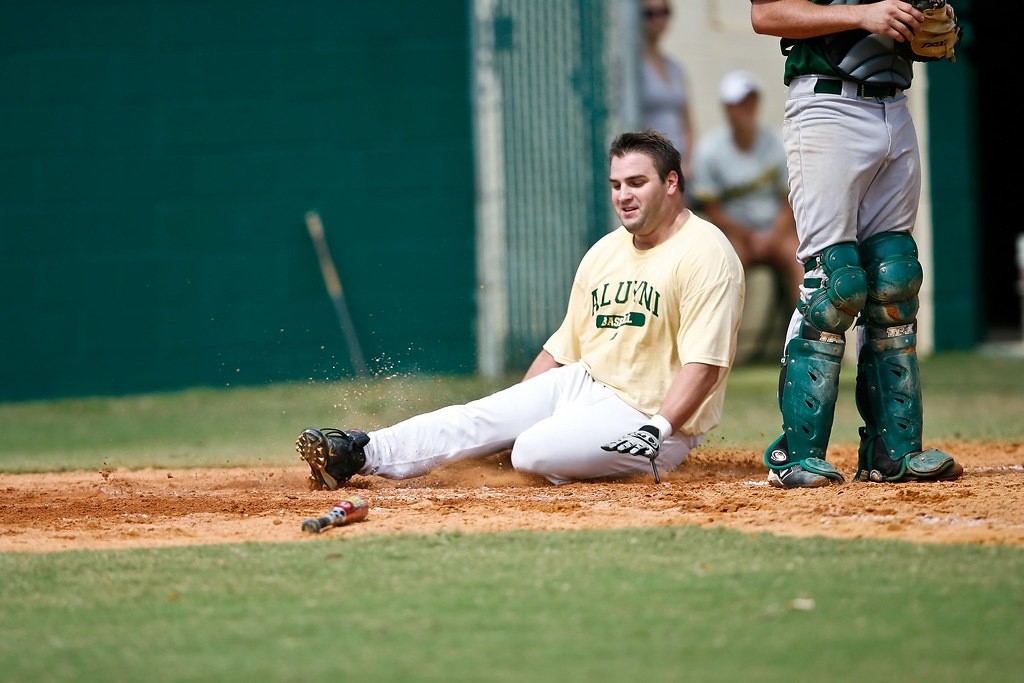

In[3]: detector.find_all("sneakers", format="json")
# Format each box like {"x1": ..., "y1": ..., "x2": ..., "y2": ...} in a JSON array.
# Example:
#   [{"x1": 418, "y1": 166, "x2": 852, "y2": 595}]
[
  {"x1": 767, "y1": 463, "x2": 829, "y2": 487},
  {"x1": 891, "y1": 463, "x2": 964, "y2": 481},
  {"x1": 295, "y1": 427, "x2": 371, "y2": 491}
]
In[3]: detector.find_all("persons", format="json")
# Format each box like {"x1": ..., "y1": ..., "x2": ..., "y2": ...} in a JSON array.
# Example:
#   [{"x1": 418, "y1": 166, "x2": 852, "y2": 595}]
[
  {"x1": 636, "y1": 0, "x2": 696, "y2": 175},
  {"x1": 296, "y1": 130, "x2": 748, "y2": 489},
  {"x1": 688, "y1": 74, "x2": 804, "y2": 315},
  {"x1": 750, "y1": 0, "x2": 955, "y2": 487}
]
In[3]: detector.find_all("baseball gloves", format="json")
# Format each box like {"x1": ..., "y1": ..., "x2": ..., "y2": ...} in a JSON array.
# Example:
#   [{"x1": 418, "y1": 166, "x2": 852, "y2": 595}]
[
  {"x1": 306, "y1": 211, "x2": 370, "y2": 380},
  {"x1": 894, "y1": 0, "x2": 963, "y2": 63}
]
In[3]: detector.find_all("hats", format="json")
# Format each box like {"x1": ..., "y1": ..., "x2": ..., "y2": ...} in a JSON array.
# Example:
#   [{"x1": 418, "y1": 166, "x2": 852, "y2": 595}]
[{"x1": 720, "y1": 72, "x2": 758, "y2": 105}]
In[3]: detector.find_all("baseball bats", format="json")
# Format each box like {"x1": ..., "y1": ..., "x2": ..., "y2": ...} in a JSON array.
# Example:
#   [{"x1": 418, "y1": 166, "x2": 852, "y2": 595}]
[{"x1": 301, "y1": 495, "x2": 369, "y2": 531}]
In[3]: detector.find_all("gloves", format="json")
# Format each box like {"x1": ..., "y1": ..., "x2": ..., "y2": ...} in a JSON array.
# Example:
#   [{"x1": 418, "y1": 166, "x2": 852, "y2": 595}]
[{"x1": 601, "y1": 425, "x2": 661, "y2": 459}]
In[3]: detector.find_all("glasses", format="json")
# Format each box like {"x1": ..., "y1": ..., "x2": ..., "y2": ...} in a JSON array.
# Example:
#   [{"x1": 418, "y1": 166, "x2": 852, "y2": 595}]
[{"x1": 641, "y1": 8, "x2": 669, "y2": 17}]
